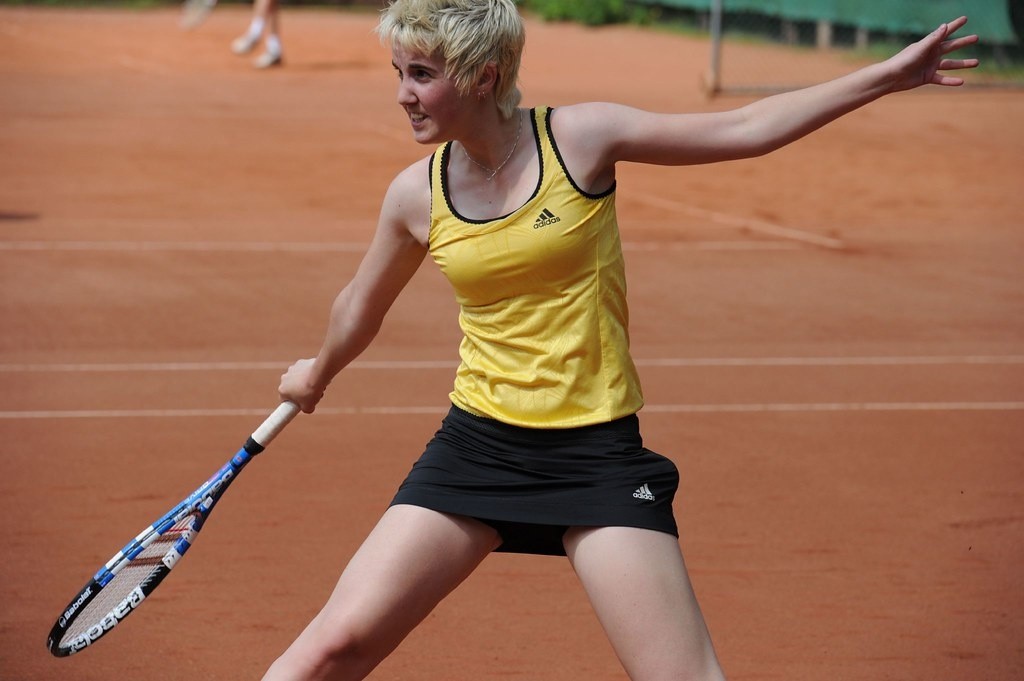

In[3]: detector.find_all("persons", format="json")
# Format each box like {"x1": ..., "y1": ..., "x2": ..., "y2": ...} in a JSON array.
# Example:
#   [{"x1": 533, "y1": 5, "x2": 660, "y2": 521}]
[
  {"x1": 251, "y1": 0, "x2": 982, "y2": 681},
  {"x1": 230, "y1": 0, "x2": 283, "y2": 70}
]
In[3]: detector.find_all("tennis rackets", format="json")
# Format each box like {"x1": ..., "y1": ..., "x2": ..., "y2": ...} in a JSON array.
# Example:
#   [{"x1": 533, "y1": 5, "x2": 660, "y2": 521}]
[{"x1": 47, "y1": 400, "x2": 300, "y2": 656}]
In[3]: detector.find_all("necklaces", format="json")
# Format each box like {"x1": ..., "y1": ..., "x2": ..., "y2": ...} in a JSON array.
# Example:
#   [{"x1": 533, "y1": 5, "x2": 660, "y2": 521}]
[{"x1": 457, "y1": 106, "x2": 523, "y2": 181}]
